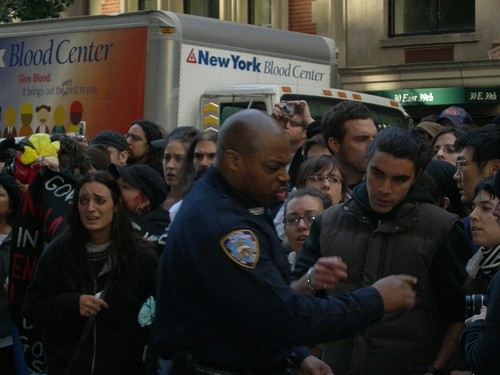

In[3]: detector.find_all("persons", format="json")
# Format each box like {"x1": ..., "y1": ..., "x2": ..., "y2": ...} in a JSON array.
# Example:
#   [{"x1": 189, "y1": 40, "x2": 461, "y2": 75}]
[
  {"x1": 288, "y1": 126, "x2": 478, "y2": 375},
  {"x1": 416, "y1": 105, "x2": 500, "y2": 375},
  {"x1": 0, "y1": 121, "x2": 218, "y2": 375},
  {"x1": 153, "y1": 108, "x2": 418, "y2": 375},
  {"x1": 25, "y1": 171, "x2": 161, "y2": 375},
  {"x1": 269, "y1": 99, "x2": 378, "y2": 274}
]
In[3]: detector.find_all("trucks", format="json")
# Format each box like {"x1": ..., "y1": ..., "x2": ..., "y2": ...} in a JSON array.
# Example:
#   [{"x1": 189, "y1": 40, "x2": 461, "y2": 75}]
[{"x1": 0, "y1": 8, "x2": 416, "y2": 149}]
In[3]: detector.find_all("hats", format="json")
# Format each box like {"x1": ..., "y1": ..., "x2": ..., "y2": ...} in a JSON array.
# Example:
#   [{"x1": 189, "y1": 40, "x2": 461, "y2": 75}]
[
  {"x1": 435, "y1": 106, "x2": 472, "y2": 128},
  {"x1": 151, "y1": 126, "x2": 184, "y2": 149},
  {"x1": 415, "y1": 121, "x2": 445, "y2": 141},
  {"x1": 109, "y1": 163, "x2": 170, "y2": 207},
  {"x1": 426, "y1": 160, "x2": 464, "y2": 209},
  {"x1": 90, "y1": 129, "x2": 129, "y2": 150}
]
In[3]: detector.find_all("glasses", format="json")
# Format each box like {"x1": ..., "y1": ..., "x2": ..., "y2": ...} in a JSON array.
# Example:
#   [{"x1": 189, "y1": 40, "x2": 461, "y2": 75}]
[
  {"x1": 309, "y1": 175, "x2": 340, "y2": 183},
  {"x1": 283, "y1": 215, "x2": 317, "y2": 226},
  {"x1": 457, "y1": 159, "x2": 475, "y2": 172}
]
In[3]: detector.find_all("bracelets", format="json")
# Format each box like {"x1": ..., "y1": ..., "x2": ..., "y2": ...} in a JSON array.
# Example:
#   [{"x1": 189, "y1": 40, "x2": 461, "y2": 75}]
[
  {"x1": 306, "y1": 266, "x2": 318, "y2": 292},
  {"x1": 423, "y1": 366, "x2": 440, "y2": 375}
]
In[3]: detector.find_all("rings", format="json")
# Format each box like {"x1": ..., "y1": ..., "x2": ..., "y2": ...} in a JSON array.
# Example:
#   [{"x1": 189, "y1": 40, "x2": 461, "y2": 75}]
[{"x1": 323, "y1": 282, "x2": 327, "y2": 289}]
